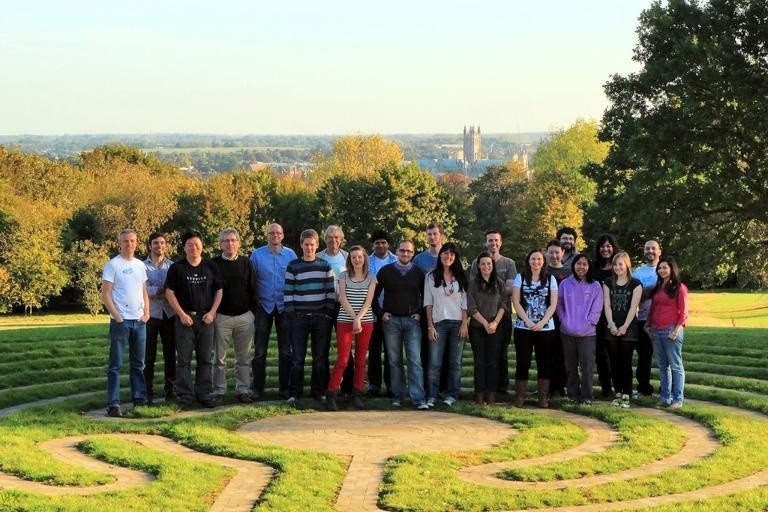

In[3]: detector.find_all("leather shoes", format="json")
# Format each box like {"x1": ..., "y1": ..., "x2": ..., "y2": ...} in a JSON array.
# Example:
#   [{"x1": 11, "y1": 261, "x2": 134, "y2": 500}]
[
  {"x1": 350, "y1": 395, "x2": 365, "y2": 409},
  {"x1": 326, "y1": 398, "x2": 338, "y2": 411}
]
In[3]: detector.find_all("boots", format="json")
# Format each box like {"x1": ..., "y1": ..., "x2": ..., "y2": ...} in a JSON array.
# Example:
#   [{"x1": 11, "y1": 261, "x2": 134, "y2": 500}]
[
  {"x1": 487, "y1": 392, "x2": 495, "y2": 407},
  {"x1": 514, "y1": 378, "x2": 527, "y2": 407},
  {"x1": 278, "y1": 381, "x2": 290, "y2": 399},
  {"x1": 145, "y1": 378, "x2": 154, "y2": 406},
  {"x1": 164, "y1": 378, "x2": 178, "y2": 403},
  {"x1": 251, "y1": 381, "x2": 265, "y2": 400},
  {"x1": 474, "y1": 391, "x2": 484, "y2": 405},
  {"x1": 537, "y1": 378, "x2": 550, "y2": 407}
]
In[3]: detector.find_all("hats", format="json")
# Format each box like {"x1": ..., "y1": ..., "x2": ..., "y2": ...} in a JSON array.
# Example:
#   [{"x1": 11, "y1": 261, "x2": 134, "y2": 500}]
[{"x1": 369, "y1": 230, "x2": 392, "y2": 243}]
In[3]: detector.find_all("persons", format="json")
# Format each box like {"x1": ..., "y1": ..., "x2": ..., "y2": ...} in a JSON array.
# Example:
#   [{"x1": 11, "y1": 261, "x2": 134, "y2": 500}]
[
  {"x1": 250, "y1": 223, "x2": 299, "y2": 401},
  {"x1": 100, "y1": 229, "x2": 152, "y2": 420},
  {"x1": 206, "y1": 226, "x2": 259, "y2": 405},
  {"x1": 162, "y1": 230, "x2": 226, "y2": 410},
  {"x1": 284, "y1": 222, "x2": 689, "y2": 417},
  {"x1": 141, "y1": 233, "x2": 180, "y2": 406}
]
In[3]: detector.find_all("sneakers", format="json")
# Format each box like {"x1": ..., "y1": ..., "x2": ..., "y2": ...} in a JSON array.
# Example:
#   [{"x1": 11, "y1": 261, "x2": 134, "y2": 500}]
[
  {"x1": 668, "y1": 402, "x2": 683, "y2": 410},
  {"x1": 442, "y1": 395, "x2": 457, "y2": 406},
  {"x1": 610, "y1": 394, "x2": 621, "y2": 407},
  {"x1": 213, "y1": 394, "x2": 224, "y2": 404},
  {"x1": 235, "y1": 394, "x2": 253, "y2": 404},
  {"x1": 365, "y1": 389, "x2": 379, "y2": 398},
  {"x1": 427, "y1": 397, "x2": 438, "y2": 406},
  {"x1": 600, "y1": 391, "x2": 616, "y2": 401},
  {"x1": 655, "y1": 402, "x2": 667, "y2": 408},
  {"x1": 107, "y1": 405, "x2": 122, "y2": 417},
  {"x1": 621, "y1": 394, "x2": 631, "y2": 408},
  {"x1": 315, "y1": 395, "x2": 329, "y2": 405},
  {"x1": 568, "y1": 398, "x2": 579, "y2": 406},
  {"x1": 580, "y1": 399, "x2": 592, "y2": 407},
  {"x1": 632, "y1": 391, "x2": 651, "y2": 401},
  {"x1": 133, "y1": 402, "x2": 144, "y2": 409},
  {"x1": 197, "y1": 397, "x2": 214, "y2": 408},
  {"x1": 385, "y1": 389, "x2": 397, "y2": 398},
  {"x1": 414, "y1": 401, "x2": 428, "y2": 409},
  {"x1": 179, "y1": 397, "x2": 193, "y2": 409},
  {"x1": 392, "y1": 399, "x2": 402, "y2": 405},
  {"x1": 286, "y1": 396, "x2": 297, "y2": 407}
]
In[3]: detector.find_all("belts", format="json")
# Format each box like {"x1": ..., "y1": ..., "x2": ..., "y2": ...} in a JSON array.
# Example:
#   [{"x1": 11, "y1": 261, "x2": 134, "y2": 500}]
[
  {"x1": 437, "y1": 319, "x2": 462, "y2": 324},
  {"x1": 184, "y1": 311, "x2": 208, "y2": 317},
  {"x1": 389, "y1": 312, "x2": 414, "y2": 318},
  {"x1": 296, "y1": 312, "x2": 328, "y2": 321}
]
